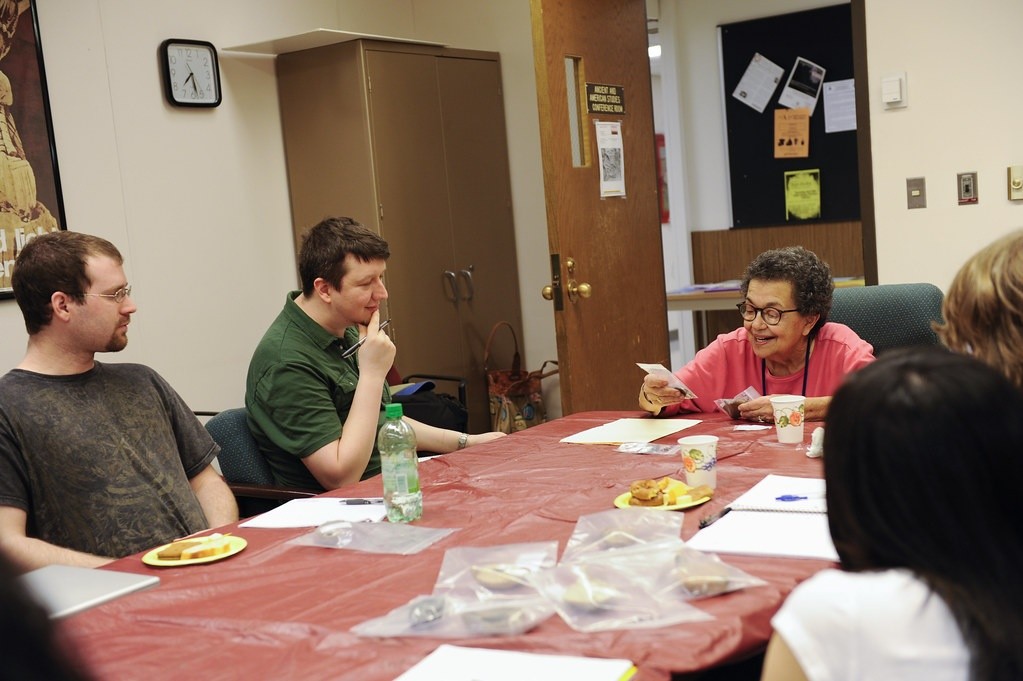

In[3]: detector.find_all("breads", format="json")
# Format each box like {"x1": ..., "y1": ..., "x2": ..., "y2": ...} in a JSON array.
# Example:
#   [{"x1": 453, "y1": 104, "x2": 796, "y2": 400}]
[
  {"x1": 181, "y1": 538, "x2": 231, "y2": 559},
  {"x1": 627, "y1": 478, "x2": 714, "y2": 506}
]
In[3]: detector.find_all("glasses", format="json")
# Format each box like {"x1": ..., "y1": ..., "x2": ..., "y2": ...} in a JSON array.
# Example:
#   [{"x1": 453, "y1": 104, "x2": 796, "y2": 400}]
[
  {"x1": 67, "y1": 285, "x2": 132, "y2": 303},
  {"x1": 736, "y1": 301, "x2": 802, "y2": 325}
]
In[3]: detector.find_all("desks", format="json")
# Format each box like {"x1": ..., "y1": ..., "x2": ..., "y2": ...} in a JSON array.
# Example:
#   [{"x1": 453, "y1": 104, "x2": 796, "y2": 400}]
[
  {"x1": 53, "y1": 411, "x2": 840, "y2": 681},
  {"x1": 665, "y1": 277, "x2": 865, "y2": 349}
]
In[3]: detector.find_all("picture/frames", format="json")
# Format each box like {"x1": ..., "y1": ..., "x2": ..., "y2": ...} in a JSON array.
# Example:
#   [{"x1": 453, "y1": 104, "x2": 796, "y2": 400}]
[{"x1": 0, "y1": 0, "x2": 66, "y2": 301}]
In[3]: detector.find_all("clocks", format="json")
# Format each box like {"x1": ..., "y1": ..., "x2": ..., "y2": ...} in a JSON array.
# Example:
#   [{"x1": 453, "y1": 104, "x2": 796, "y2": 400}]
[{"x1": 160, "y1": 39, "x2": 222, "y2": 107}]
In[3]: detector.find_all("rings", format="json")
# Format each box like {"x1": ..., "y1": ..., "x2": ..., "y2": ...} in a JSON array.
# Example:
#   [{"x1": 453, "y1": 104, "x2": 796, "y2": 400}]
[
  {"x1": 657, "y1": 396, "x2": 662, "y2": 403},
  {"x1": 759, "y1": 416, "x2": 764, "y2": 423}
]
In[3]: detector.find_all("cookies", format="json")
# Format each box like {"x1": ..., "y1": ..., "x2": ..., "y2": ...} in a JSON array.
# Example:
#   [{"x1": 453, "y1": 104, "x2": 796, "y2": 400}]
[
  {"x1": 467, "y1": 527, "x2": 729, "y2": 633},
  {"x1": 157, "y1": 542, "x2": 204, "y2": 559}
]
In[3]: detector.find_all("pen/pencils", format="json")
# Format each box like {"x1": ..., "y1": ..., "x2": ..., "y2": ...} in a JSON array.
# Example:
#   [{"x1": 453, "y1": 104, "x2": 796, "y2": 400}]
[
  {"x1": 339, "y1": 498, "x2": 383, "y2": 505},
  {"x1": 775, "y1": 495, "x2": 808, "y2": 502}
]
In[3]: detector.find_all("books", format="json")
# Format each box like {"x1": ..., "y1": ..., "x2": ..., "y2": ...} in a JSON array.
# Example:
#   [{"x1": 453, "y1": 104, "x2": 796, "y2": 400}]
[
  {"x1": 686, "y1": 474, "x2": 841, "y2": 561},
  {"x1": 241, "y1": 497, "x2": 388, "y2": 527}
]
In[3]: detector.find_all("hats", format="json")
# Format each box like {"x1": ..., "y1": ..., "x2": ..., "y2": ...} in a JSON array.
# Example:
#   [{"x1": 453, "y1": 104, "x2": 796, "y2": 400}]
[{"x1": 391, "y1": 381, "x2": 436, "y2": 396}]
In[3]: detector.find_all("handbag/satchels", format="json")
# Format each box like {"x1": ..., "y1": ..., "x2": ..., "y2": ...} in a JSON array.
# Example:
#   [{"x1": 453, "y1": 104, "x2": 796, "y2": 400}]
[
  {"x1": 484, "y1": 321, "x2": 558, "y2": 434},
  {"x1": 392, "y1": 394, "x2": 468, "y2": 433}
]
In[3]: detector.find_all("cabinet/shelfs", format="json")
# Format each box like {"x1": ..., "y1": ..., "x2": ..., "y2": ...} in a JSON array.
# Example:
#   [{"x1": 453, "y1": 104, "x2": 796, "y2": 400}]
[{"x1": 276, "y1": 39, "x2": 527, "y2": 436}]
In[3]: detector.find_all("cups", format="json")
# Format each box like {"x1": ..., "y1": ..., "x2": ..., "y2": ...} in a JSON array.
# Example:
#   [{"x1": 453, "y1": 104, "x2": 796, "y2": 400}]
[
  {"x1": 771, "y1": 395, "x2": 805, "y2": 444},
  {"x1": 677, "y1": 434, "x2": 720, "y2": 490}
]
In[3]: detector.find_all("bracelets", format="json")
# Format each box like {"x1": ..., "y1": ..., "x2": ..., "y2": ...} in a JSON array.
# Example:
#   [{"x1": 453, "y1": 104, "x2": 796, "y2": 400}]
[
  {"x1": 641, "y1": 384, "x2": 652, "y2": 404},
  {"x1": 457, "y1": 433, "x2": 470, "y2": 450}
]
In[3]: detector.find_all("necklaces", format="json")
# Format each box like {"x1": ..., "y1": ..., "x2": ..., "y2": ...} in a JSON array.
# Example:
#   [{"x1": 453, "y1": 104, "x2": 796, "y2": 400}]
[{"x1": 762, "y1": 340, "x2": 810, "y2": 396}]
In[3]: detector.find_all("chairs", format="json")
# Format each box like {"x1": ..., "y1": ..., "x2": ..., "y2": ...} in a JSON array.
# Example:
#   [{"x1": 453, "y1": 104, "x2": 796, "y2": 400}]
[{"x1": 828, "y1": 284, "x2": 947, "y2": 355}]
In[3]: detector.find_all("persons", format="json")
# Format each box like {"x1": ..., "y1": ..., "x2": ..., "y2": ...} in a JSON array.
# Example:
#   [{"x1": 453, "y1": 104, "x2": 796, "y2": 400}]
[
  {"x1": 931, "y1": 230, "x2": 1023, "y2": 390},
  {"x1": 245, "y1": 216, "x2": 508, "y2": 505},
  {"x1": 638, "y1": 248, "x2": 876, "y2": 423},
  {"x1": 0, "y1": 231, "x2": 239, "y2": 576},
  {"x1": 760, "y1": 347, "x2": 1023, "y2": 681}
]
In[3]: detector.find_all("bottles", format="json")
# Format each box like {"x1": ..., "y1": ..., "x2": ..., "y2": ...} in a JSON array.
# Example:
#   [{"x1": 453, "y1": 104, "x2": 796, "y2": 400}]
[{"x1": 377, "y1": 403, "x2": 423, "y2": 524}]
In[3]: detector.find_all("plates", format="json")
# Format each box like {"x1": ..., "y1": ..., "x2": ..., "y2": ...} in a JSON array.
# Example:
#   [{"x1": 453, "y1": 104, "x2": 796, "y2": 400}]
[
  {"x1": 614, "y1": 484, "x2": 712, "y2": 511},
  {"x1": 141, "y1": 535, "x2": 248, "y2": 566}
]
